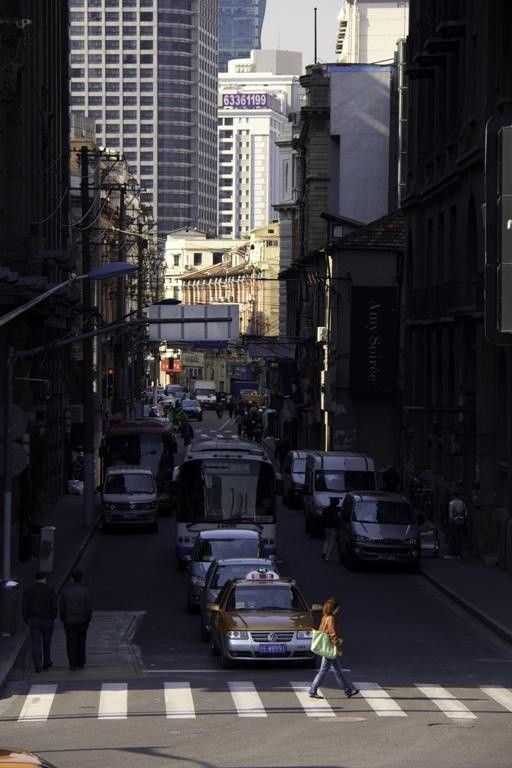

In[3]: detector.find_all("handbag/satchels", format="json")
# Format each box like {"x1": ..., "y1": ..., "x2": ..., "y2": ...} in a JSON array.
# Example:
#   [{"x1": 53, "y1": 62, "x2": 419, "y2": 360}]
[{"x1": 311, "y1": 629, "x2": 344, "y2": 660}]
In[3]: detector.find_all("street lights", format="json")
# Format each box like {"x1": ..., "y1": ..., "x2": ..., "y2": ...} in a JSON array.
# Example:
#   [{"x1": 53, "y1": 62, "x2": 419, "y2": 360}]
[{"x1": 0, "y1": 261, "x2": 182, "y2": 440}]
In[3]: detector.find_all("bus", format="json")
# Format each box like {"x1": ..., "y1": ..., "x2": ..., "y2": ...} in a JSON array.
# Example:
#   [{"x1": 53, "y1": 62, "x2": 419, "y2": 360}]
[
  {"x1": 97, "y1": 419, "x2": 178, "y2": 516},
  {"x1": 171, "y1": 437, "x2": 281, "y2": 568}
]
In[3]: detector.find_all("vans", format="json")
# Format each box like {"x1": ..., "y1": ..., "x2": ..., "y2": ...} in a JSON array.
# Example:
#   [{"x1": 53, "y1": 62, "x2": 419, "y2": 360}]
[{"x1": 302, "y1": 451, "x2": 379, "y2": 540}]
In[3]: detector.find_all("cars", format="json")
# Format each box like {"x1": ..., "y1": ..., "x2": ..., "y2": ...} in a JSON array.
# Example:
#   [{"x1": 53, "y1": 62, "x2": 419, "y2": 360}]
[
  {"x1": 0, "y1": 746, "x2": 60, "y2": 767},
  {"x1": 199, "y1": 557, "x2": 282, "y2": 642},
  {"x1": 204, "y1": 567, "x2": 324, "y2": 666},
  {"x1": 141, "y1": 379, "x2": 258, "y2": 422}
]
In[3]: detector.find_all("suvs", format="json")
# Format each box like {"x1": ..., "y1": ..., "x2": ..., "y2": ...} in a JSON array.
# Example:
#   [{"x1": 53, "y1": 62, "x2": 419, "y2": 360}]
[
  {"x1": 95, "y1": 464, "x2": 164, "y2": 536},
  {"x1": 337, "y1": 490, "x2": 423, "y2": 574},
  {"x1": 183, "y1": 527, "x2": 276, "y2": 617},
  {"x1": 281, "y1": 450, "x2": 309, "y2": 512}
]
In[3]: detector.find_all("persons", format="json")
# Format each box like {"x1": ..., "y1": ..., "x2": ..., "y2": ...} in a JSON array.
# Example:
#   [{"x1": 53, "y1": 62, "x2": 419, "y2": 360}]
[
  {"x1": 181, "y1": 421, "x2": 194, "y2": 446},
  {"x1": 443, "y1": 492, "x2": 465, "y2": 559},
  {"x1": 149, "y1": 400, "x2": 181, "y2": 417},
  {"x1": 309, "y1": 598, "x2": 360, "y2": 700},
  {"x1": 383, "y1": 465, "x2": 400, "y2": 492},
  {"x1": 22, "y1": 572, "x2": 58, "y2": 672},
  {"x1": 274, "y1": 440, "x2": 289, "y2": 473},
  {"x1": 321, "y1": 498, "x2": 340, "y2": 561},
  {"x1": 216, "y1": 392, "x2": 263, "y2": 442},
  {"x1": 59, "y1": 570, "x2": 92, "y2": 671}
]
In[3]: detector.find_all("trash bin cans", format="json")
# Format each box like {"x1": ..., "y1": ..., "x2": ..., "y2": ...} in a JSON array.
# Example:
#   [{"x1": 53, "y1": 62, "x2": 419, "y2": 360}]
[{"x1": 0, "y1": 579, "x2": 19, "y2": 639}]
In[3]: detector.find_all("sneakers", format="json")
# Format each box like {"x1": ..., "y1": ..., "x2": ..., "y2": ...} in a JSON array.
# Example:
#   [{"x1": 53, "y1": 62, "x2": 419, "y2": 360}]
[{"x1": 322, "y1": 557, "x2": 335, "y2": 561}]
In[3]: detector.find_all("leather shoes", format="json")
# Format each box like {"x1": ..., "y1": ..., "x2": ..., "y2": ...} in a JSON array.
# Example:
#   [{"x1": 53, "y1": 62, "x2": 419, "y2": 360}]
[
  {"x1": 311, "y1": 694, "x2": 324, "y2": 698},
  {"x1": 43, "y1": 662, "x2": 52, "y2": 669},
  {"x1": 348, "y1": 690, "x2": 359, "y2": 697}
]
